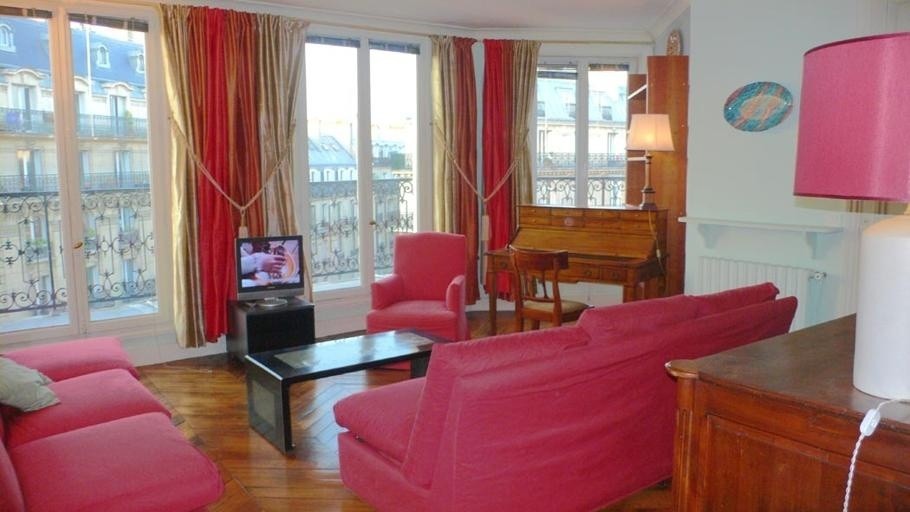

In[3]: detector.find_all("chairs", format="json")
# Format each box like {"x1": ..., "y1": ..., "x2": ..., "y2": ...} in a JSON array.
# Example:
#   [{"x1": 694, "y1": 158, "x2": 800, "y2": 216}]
[
  {"x1": 366, "y1": 232, "x2": 468, "y2": 369},
  {"x1": 507, "y1": 244, "x2": 593, "y2": 332}
]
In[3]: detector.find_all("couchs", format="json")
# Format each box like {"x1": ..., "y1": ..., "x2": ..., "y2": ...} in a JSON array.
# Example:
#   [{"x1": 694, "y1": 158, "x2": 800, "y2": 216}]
[
  {"x1": 0, "y1": 333, "x2": 225, "y2": 512},
  {"x1": 334, "y1": 298, "x2": 800, "y2": 512}
]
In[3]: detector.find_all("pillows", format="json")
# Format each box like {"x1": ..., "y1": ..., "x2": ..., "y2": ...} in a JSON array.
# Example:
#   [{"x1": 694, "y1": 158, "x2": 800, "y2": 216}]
[
  {"x1": 0, "y1": 357, "x2": 61, "y2": 412},
  {"x1": 400, "y1": 283, "x2": 780, "y2": 486}
]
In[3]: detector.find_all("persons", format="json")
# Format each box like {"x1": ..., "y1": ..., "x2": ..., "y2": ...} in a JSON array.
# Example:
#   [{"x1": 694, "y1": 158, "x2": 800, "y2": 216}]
[
  {"x1": 239, "y1": 243, "x2": 259, "y2": 281},
  {"x1": 240, "y1": 250, "x2": 288, "y2": 287}
]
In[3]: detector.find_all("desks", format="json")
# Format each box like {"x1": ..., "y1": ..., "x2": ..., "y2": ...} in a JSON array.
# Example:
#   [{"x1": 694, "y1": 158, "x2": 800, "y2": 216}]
[{"x1": 665, "y1": 312, "x2": 910, "y2": 512}]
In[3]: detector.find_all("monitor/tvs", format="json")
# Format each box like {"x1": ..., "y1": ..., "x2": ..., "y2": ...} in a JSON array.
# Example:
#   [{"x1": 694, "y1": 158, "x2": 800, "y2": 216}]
[{"x1": 234, "y1": 234, "x2": 306, "y2": 310}]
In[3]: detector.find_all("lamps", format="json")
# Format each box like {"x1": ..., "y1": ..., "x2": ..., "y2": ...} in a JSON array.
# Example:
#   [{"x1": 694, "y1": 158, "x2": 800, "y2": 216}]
[
  {"x1": 623, "y1": 113, "x2": 675, "y2": 209},
  {"x1": 791, "y1": 32, "x2": 910, "y2": 404}
]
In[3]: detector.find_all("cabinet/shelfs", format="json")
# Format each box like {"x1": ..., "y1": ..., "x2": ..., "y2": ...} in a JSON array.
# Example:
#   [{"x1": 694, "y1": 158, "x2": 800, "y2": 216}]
[
  {"x1": 623, "y1": 56, "x2": 689, "y2": 302},
  {"x1": 226, "y1": 298, "x2": 314, "y2": 363}
]
